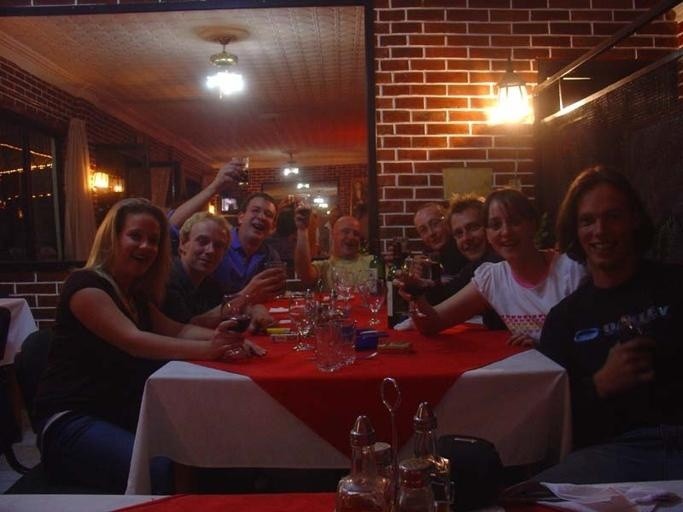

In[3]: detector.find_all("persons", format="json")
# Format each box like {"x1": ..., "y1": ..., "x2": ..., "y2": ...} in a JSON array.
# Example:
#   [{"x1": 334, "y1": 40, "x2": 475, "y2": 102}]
[
  {"x1": 403, "y1": 185, "x2": 593, "y2": 352},
  {"x1": 395, "y1": 195, "x2": 509, "y2": 330},
  {"x1": 533, "y1": 162, "x2": 683, "y2": 481},
  {"x1": 167, "y1": 158, "x2": 284, "y2": 326},
  {"x1": 23, "y1": 198, "x2": 268, "y2": 493},
  {"x1": 392, "y1": 202, "x2": 469, "y2": 307},
  {"x1": 290, "y1": 200, "x2": 382, "y2": 295},
  {"x1": 155, "y1": 210, "x2": 289, "y2": 337}
]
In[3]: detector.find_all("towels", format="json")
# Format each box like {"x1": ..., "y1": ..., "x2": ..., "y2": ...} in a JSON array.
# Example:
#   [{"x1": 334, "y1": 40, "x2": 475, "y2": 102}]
[{"x1": 531, "y1": 479, "x2": 683, "y2": 512}]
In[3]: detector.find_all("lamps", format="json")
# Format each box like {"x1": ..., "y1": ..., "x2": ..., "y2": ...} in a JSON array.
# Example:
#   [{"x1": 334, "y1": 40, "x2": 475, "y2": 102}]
[
  {"x1": 280, "y1": 146, "x2": 333, "y2": 214},
  {"x1": 490, "y1": 55, "x2": 536, "y2": 129},
  {"x1": 195, "y1": 25, "x2": 251, "y2": 101}
]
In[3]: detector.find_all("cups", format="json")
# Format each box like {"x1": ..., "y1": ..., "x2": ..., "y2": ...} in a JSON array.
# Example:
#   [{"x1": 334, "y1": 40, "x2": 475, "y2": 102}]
[
  {"x1": 232, "y1": 155, "x2": 249, "y2": 186},
  {"x1": 297, "y1": 202, "x2": 311, "y2": 228},
  {"x1": 292, "y1": 264, "x2": 385, "y2": 370},
  {"x1": 616, "y1": 313, "x2": 648, "y2": 343},
  {"x1": 263, "y1": 260, "x2": 287, "y2": 300}
]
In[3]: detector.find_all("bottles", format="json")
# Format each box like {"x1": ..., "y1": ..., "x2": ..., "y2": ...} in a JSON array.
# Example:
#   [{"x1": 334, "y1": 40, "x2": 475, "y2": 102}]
[
  {"x1": 366, "y1": 247, "x2": 384, "y2": 295},
  {"x1": 336, "y1": 400, "x2": 455, "y2": 512},
  {"x1": 385, "y1": 242, "x2": 405, "y2": 326}
]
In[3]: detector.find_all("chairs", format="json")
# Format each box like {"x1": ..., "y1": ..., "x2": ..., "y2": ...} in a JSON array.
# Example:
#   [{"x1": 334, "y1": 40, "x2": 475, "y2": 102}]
[{"x1": 0, "y1": 294, "x2": 123, "y2": 495}]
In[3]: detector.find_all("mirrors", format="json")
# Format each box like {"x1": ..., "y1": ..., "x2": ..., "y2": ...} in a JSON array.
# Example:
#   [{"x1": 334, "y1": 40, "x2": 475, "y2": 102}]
[{"x1": 258, "y1": 177, "x2": 341, "y2": 217}]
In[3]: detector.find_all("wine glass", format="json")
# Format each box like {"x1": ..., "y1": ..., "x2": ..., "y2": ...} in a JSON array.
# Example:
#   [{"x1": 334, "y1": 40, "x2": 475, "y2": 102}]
[
  {"x1": 403, "y1": 258, "x2": 431, "y2": 320},
  {"x1": 220, "y1": 295, "x2": 253, "y2": 360}
]
